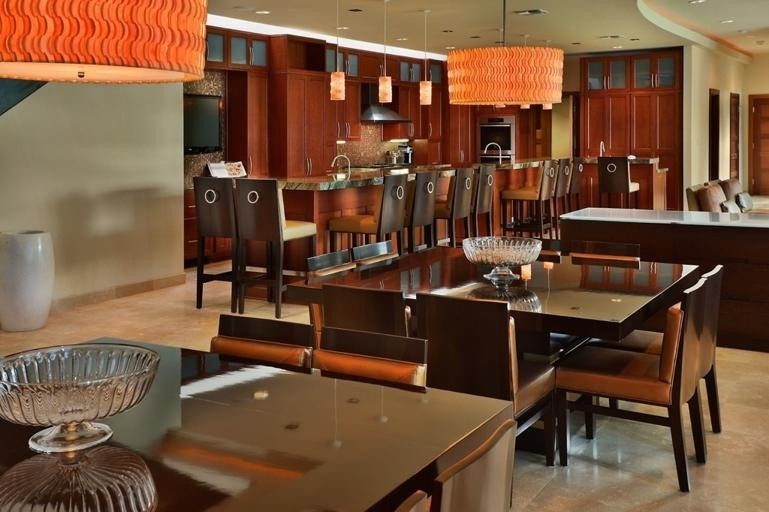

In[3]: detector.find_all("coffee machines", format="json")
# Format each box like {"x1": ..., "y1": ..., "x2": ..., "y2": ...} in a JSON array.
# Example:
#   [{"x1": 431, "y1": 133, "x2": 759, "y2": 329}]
[{"x1": 398, "y1": 145, "x2": 414, "y2": 165}]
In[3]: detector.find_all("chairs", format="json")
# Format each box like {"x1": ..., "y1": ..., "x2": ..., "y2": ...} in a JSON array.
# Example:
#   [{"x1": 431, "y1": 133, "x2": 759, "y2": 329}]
[
  {"x1": 535, "y1": 241, "x2": 562, "y2": 257},
  {"x1": 554, "y1": 278, "x2": 709, "y2": 493},
  {"x1": 414, "y1": 289, "x2": 559, "y2": 475},
  {"x1": 302, "y1": 247, "x2": 356, "y2": 348},
  {"x1": 585, "y1": 263, "x2": 725, "y2": 459},
  {"x1": 570, "y1": 239, "x2": 640, "y2": 261},
  {"x1": 686, "y1": 177, "x2": 769, "y2": 214},
  {"x1": 209, "y1": 314, "x2": 314, "y2": 377},
  {"x1": 316, "y1": 328, "x2": 431, "y2": 392},
  {"x1": 320, "y1": 282, "x2": 411, "y2": 338},
  {"x1": 350, "y1": 239, "x2": 402, "y2": 270}
]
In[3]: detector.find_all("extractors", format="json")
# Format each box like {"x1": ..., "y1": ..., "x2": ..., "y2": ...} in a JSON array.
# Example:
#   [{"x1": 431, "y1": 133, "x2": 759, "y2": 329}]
[{"x1": 361, "y1": 84, "x2": 410, "y2": 121}]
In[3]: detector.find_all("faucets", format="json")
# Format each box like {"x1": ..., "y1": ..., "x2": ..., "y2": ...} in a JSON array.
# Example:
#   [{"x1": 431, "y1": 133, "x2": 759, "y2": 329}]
[
  {"x1": 484, "y1": 142, "x2": 502, "y2": 166},
  {"x1": 600, "y1": 141, "x2": 605, "y2": 157},
  {"x1": 331, "y1": 155, "x2": 351, "y2": 176}
]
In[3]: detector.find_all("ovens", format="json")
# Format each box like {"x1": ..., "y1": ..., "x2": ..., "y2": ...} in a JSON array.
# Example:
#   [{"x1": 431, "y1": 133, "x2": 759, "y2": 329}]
[{"x1": 479, "y1": 116, "x2": 514, "y2": 163}]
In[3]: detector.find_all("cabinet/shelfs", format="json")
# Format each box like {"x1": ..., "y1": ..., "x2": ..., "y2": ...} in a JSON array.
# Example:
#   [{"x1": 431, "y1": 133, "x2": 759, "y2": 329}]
[
  {"x1": 268, "y1": 73, "x2": 474, "y2": 178},
  {"x1": 581, "y1": 90, "x2": 683, "y2": 212}
]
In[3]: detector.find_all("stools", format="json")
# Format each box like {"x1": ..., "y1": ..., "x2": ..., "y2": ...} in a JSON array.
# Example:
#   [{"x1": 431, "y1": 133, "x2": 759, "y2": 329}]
[
  {"x1": 431, "y1": 166, "x2": 479, "y2": 247},
  {"x1": 234, "y1": 176, "x2": 318, "y2": 316},
  {"x1": 466, "y1": 162, "x2": 497, "y2": 236},
  {"x1": 191, "y1": 173, "x2": 265, "y2": 312},
  {"x1": 385, "y1": 171, "x2": 440, "y2": 254},
  {"x1": 498, "y1": 157, "x2": 588, "y2": 238},
  {"x1": 325, "y1": 173, "x2": 409, "y2": 263}
]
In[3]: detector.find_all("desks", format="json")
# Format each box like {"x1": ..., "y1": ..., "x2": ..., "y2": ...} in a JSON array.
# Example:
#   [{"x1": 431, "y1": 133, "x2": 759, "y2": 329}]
[
  {"x1": 0, "y1": 337, "x2": 515, "y2": 511},
  {"x1": 284, "y1": 242, "x2": 700, "y2": 457}
]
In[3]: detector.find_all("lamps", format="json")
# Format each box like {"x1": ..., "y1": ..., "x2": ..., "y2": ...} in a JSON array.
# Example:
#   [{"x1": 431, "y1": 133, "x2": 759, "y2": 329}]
[
  {"x1": 419, "y1": 9, "x2": 432, "y2": 105},
  {"x1": 445, "y1": 1, "x2": 564, "y2": 106},
  {"x1": 330, "y1": 0, "x2": 346, "y2": 100},
  {"x1": 0, "y1": 1, "x2": 208, "y2": 86},
  {"x1": 494, "y1": 28, "x2": 553, "y2": 111},
  {"x1": 379, "y1": 1, "x2": 393, "y2": 103}
]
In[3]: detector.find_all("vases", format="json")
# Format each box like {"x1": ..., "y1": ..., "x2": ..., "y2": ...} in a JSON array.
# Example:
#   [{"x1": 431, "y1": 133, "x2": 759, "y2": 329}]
[{"x1": 0, "y1": 229, "x2": 54, "y2": 332}]
